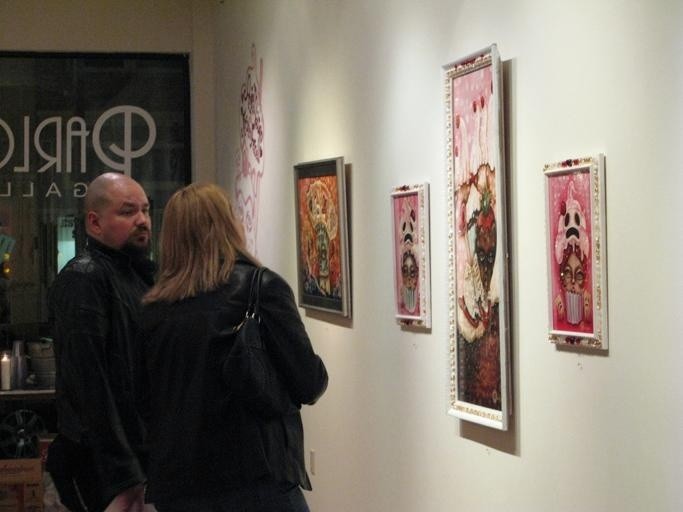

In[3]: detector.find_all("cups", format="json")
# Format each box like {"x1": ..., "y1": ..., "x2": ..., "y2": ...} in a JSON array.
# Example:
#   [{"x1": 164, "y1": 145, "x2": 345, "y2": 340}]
[{"x1": 11, "y1": 341, "x2": 28, "y2": 389}]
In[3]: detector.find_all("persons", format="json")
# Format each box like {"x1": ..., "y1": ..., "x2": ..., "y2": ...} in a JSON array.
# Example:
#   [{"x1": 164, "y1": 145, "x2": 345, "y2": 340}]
[
  {"x1": 129, "y1": 176, "x2": 330, "y2": 512},
  {"x1": 44, "y1": 171, "x2": 168, "y2": 512}
]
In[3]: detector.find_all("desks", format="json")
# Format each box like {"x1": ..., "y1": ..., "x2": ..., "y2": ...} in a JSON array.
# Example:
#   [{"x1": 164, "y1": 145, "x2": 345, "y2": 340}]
[{"x1": 0, "y1": 388, "x2": 56, "y2": 402}]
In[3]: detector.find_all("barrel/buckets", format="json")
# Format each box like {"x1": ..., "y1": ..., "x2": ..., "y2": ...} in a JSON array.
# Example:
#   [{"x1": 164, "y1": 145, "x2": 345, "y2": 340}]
[{"x1": 26, "y1": 336, "x2": 56, "y2": 387}]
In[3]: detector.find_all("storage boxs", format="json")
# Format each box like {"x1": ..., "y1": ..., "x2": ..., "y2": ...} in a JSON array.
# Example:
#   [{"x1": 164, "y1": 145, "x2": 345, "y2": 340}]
[{"x1": 0, "y1": 459, "x2": 44, "y2": 512}]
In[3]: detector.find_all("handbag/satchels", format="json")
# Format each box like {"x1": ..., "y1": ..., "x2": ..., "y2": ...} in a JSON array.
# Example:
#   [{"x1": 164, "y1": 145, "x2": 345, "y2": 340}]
[{"x1": 219, "y1": 267, "x2": 290, "y2": 418}]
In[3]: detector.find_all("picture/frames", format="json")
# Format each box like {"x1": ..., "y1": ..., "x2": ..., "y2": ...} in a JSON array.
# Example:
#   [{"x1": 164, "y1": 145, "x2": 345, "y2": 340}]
[
  {"x1": 441, "y1": 43, "x2": 515, "y2": 435},
  {"x1": 294, "y1": 156, "x2": 353, "y2": 320},
  {"x1": 544, "y1": 153, "x2": 607, "y2": 353},
  {"x1": 390, "y1": 182, "x2": 431, "y2": 329}
]
